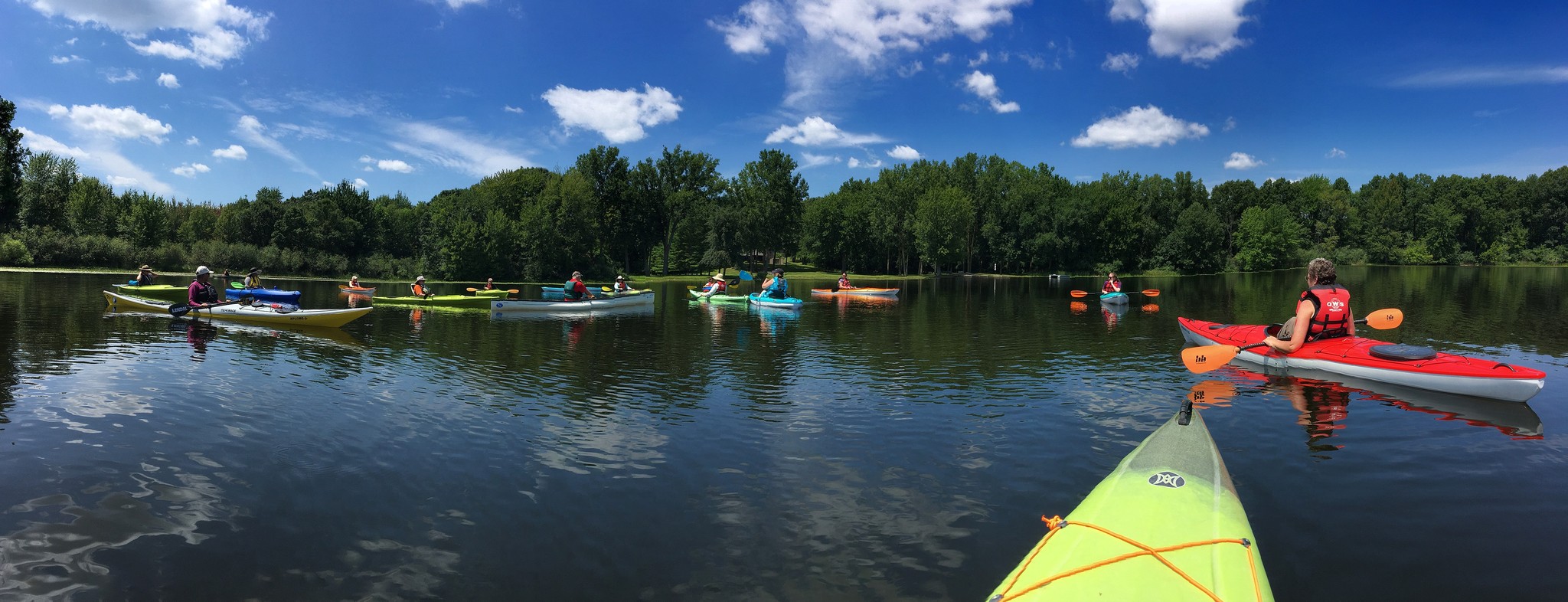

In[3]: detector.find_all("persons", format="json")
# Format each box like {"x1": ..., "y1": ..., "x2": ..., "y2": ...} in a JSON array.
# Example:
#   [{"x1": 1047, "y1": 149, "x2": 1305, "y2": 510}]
[
  {"x1": 564, "y1": 271, "x2": 597, "y2": 301},
  {"x1": 1262, "y1": 258, "x2": 1356, "y2": 353},
  {"x1": 414, "y1": 276, "x2": 433, "y2": 297},
  {"x1": 244, "y1": 267, "x2": 265, "y2": 289},
  {"x1": 136, "y1": 265, "x2": 159, "y2": 286},
  {"x1": 704, "y1": 278, "x2": 715, "y2": 287},
  {"x1": 614, "y1": 276, "x2": 635, "y2": 292},
  {"x1": 703, "y1": 273, "x2": 727, "y2": 298},
  {"x1": 349, "y1": 276, "x2": 360, "y2": 289},
  {"x1": 189, "y1": 266, "x2": 231, "y2": 309},
  {"x1": 1102, "y1": 273, "x2": 1122, "y2": 294},
  {"x1": 483, "y1": 278, "x2": 499, "y2": 290},
  {"x1": 759, "y1": 268, "x2": 788, "y2": 300},
  {"x1": 224, "y1": 269, "x2": 232, "y2": 276},
  {"x1": 837, "y1": 272, "x2": 856, "y2": 289}
]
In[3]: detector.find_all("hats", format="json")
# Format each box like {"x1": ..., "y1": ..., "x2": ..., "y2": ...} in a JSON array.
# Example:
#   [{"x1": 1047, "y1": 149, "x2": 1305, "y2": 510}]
[
  {"x1": 712, "y1": 273, "x2": 725, "y2": 281},
  {"x1": 195, "y1": 266, "x2": 214, "y2": 276},
  {"x1": 139, "y1": 265, "x2": 153, "y2": 270},
  {"x1": 772, "y1": 268, "x2": 784, "y2": 274},
  {"x1": 616, "y1": 276, "x2": 624, "y2": 282},
  {"x1": 352, "y1": 276, "x2": 358, "y2": 279},
  {"x1": 572, "y1": 271, "x2": 583, "y2": 277},
  {"x1": 488, "y1": 278, "x2": 493, "y2": 281},
  {"x1": 248, "y1": 267, "x2": 262, "y2": 276},
  {"x1": 414, "y1": 275, "x2": 428, "y2": 283}
]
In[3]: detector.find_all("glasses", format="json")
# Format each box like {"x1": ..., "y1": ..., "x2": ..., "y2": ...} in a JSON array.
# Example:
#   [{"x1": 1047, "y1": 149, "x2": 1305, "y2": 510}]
[
  {"x1": 355, "y1": 279, "x2": 357, "y2": 279},
  {"x1": 1305, "y1": 276, "x2": 1310, "y2": 281},
  {"x1": 207, "y1": 273, "x2": 210, "y2": 276},
  {"x1": 711, "y1": 280, "x2": 713, "y2": 280},
  {"x1": 619, "y1": 279, "x2": 622, "y2": 280},
  {"x1": 1108, "y1": 276, "x2": 1113, "y2": 279},
  {"x1": 146, "y1": 270, "x2": 150, "y2": 272},
  {"x1": 843, "y1": 275, "x2": 846, "y2": 277},
  {"x1": 422, "y1": 280, "x2": 425, "y2": 282}
]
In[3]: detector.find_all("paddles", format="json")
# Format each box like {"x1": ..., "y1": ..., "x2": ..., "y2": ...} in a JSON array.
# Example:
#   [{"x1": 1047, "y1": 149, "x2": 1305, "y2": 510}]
[
  {"x1": 1070, "y1": 289, "x2": 1160, "y2": 297},
  {"x1": 739, "y1": 270, "x2": 791, "y2": 298},
  {"x1": 231, "y1": 282, "x2": 260, "y2": 289},
  {"x1": 424, "y1": 294, "x2": 443, "y2": 300},
  {"x1": 1181, "y1": 308, "x2": 1404, "y2": 374},
  {"x1": 602, "y1": 287, "x2": 652, "y2": 291},
  {"x1": 687, "y1": 278, "x2": 740, "y2": 301},
  {"x1": 112, "y1": 284, "x2": 189, "y2": 287},
  {"x1": 687, "y1": 284, "x2": 738, "y2": 290},
  {"x1": 466, "y1": 288, "x2": 519, "y2": 293},
  {"x1": 168, "y1": 294, "x2": 256, "y2": 317},
  {"x1": 338, "y1": 285, "x2": 354, "y2": 288},
  {"x1": 1070, "y1": 301, "x2": 1160, "y2": 312},
  {"x1": 831, "y1": 285, "x2": 872, "y2": 293}
]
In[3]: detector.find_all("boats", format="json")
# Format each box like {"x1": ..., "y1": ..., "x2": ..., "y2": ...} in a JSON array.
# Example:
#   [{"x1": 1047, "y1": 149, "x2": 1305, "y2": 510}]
[
  {"x1": 372, "y1": 294, "x2": 500, "y2": 310},
  {"x1": 338, "y1": 285, "x2": 377, "y2": 294},
  {"x1": 811, "y1": 287, "x2": 900, "y2": 295},
  {"x1": 225, "y1": 288, "x2": 301, "y2": 302},
  {"x1": 112, "y1": 284, "x2": 190, "y2": 297},
  {"x1": 476, "y1": 289, "x2": 509, "y2": 298},
  {"x1": 103, "y1": 290, "x2": 374, "y2": 327},
  {"x1": 1213, "y1": 357, "x2": 1546, "y2": 443},
  {"x1": 689, "y1": 289, "x2": 750, "y2": 304},
  {"x1": 491, "y1": 291, "x2": 655, "y2": 310},
  {"x1": 1099, "y1": 292, "x2": 1130, "y2": 304},
  {"x1": 1176, "y1": 315, "x2": 1548, "y2": 403},
  {"x1": 212, "y1": 275, "x2": 246, "y2": 279},
  {"x1": 541, "y1": 287, "x2": 602, "y2": 291},
  {"x1": 749, "y1": 292, "x2": 804, "y2": 309}
]
